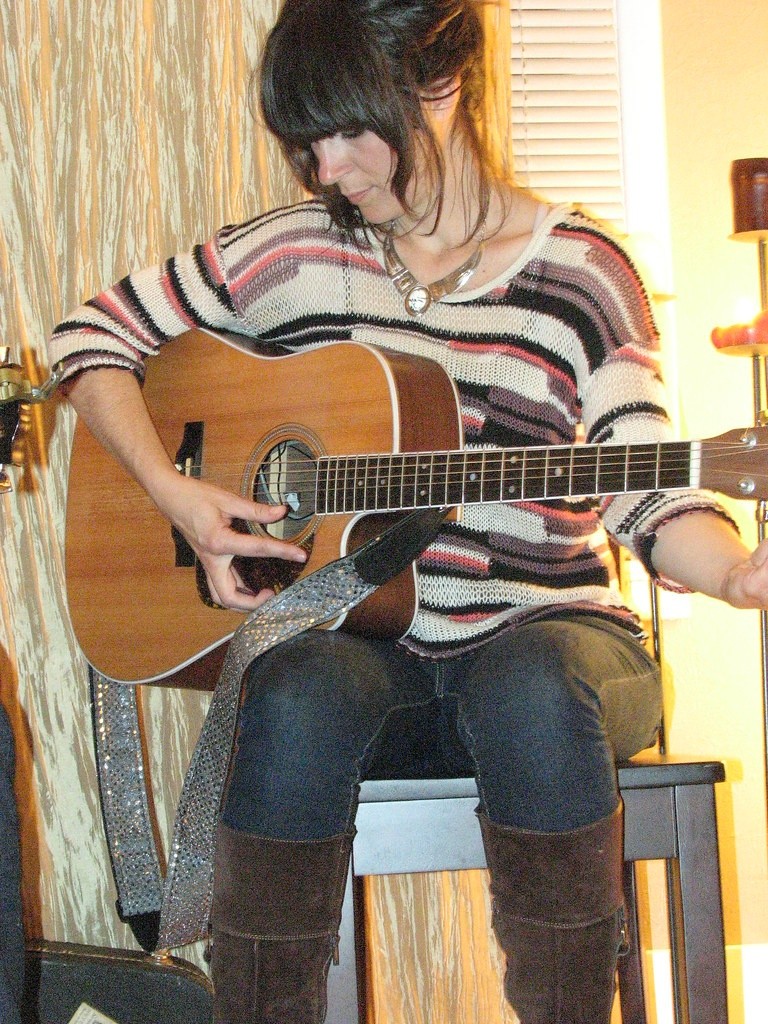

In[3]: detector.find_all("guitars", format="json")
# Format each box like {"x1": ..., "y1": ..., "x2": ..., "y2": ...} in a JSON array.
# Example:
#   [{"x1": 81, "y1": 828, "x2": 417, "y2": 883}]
[
  {"x1": 0, "y1": 397, "x2": 32, "y2": 496},
  {"x1": 58, "y1": 320, "x2": 768, "y2": 695}
]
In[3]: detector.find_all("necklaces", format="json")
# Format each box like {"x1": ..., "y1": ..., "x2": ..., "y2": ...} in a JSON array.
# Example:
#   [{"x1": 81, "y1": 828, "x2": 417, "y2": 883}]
[{"x1": 382, "y1": 220, "x2": 487, "y2": 318}]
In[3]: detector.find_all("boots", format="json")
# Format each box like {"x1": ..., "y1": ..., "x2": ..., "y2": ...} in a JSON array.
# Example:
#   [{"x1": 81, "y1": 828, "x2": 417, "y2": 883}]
[
  {"x1": 474, "y1": 797, "x2": 630, "y2": 1024},
  {"x1": 211, "y1": 816, "x2": 358, "y2": 1024}
]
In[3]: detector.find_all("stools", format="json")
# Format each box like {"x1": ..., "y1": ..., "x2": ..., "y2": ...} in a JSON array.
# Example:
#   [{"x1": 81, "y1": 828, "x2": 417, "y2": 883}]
[{"x1": 322, "y1": 761, "x2": 725, "y2": 1024}]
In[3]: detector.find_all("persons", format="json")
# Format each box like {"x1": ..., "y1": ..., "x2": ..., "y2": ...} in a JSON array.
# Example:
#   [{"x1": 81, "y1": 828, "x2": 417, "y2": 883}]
[{"x1": 48, "y1": 0, "x2": 768, "y2": 1024}]
